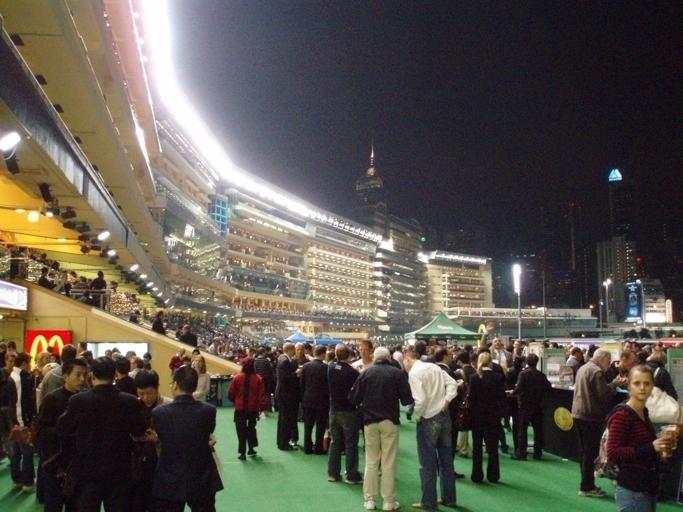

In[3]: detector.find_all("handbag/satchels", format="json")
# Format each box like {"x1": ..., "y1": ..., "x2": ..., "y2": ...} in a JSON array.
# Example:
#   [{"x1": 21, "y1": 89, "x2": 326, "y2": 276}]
[{"x1": 454, "y1": 400, "x2": 473, "y2": 431}]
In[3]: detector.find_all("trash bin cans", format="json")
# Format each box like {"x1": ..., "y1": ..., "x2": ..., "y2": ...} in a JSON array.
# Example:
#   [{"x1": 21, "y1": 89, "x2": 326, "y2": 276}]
[
  {"x1": 220, "y1": 375, "x2": 234, "y2": 407},
  {"x1": 206, "y1": 377, "x2": 220, "y2": 406}
]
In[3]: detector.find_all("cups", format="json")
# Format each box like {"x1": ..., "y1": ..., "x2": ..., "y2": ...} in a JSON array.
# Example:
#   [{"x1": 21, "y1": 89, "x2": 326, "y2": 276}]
[
  {"x1": 659, "y1": 424, "x2": 679, "y2": 450},
  {"x1": 655, "y1": 431, "x2": 673, "y2": 459}
]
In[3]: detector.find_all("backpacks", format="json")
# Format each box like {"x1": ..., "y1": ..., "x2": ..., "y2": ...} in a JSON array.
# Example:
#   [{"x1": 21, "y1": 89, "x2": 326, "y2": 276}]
[{"x1": 593, "y1": 426, "x2": 621, "y2": 479}]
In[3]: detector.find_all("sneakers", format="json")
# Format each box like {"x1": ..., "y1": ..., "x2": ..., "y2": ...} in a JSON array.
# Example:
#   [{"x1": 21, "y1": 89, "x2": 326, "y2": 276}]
[
  {"x1": 364, "y1": 501, "x2": 376, "y2": 510},
  {"x1": 382, "y1": 501, "x2": 400, "y2": 511},
  {"x1": 345, "y1": 475, "x2": 364, "y2": 484},
  {"x1": 248, "y1": 450, "x2": 257, "y2": 454},
  {"x1": 238, "y1": 455, "x2": 245, "y2": 459},
  {"x1": 578, "y1": 485, "x2": 607, "y2": 497},
  {"x1": 328, "y1": 475, "x2": 342, "y2": 481}
]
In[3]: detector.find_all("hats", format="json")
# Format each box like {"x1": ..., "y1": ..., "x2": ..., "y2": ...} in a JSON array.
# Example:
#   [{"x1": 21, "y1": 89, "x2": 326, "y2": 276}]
[{"x1": 242, "y1": 358, "x2": 255, "y2": 365}]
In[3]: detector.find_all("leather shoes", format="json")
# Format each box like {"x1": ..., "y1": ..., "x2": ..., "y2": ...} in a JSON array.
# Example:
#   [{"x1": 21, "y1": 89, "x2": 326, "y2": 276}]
[
  {"x1": 412, "y1": 503, "x2": 423, "y2": 508},
  {"x1": 437, "y1": 498, "x2": 456, "y2": 506},
  {"x1": 510, "y1": 454, "x2": 528, "y2": 460},
  {"x1": 454, "y1": 472, "x2": 465, "y2": 478}
]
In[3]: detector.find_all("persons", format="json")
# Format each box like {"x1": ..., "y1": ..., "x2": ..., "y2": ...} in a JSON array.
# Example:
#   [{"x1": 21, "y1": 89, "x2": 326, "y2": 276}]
[
  {"x1": 225, "y1": 313, "x2": 683, "y2": 511},
  {"x1": 1, "y1": 239, "x2": 224, "y2": 512}
]
